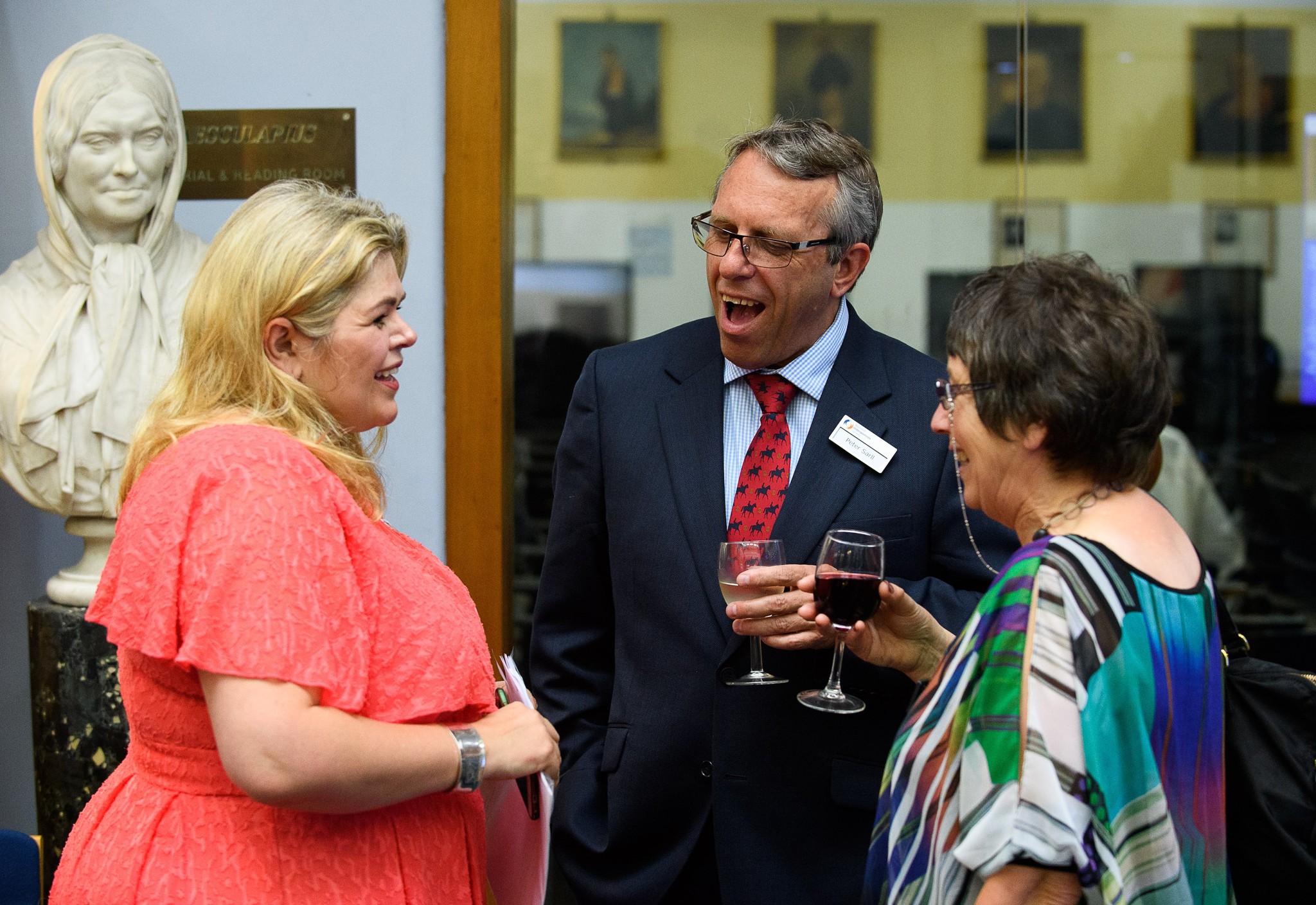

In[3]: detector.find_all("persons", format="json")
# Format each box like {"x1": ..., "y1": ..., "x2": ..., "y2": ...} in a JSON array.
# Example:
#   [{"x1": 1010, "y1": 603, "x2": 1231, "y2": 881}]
[
  {"x1": 1205, "y1": 332, "x2": 1316, "y2": 616},
  {"x1": 528, "y1": 116, "x2": 1022, "y2": 905},
  {"x1": 796, "y1": 250, "x2": 1237, "y2": 905},
  {"x1": 0, "y1": 33, "x2": 210, "y2": 607},
  {"x1": 47, "y1": 178, "x2": 562, "y2": 905}
]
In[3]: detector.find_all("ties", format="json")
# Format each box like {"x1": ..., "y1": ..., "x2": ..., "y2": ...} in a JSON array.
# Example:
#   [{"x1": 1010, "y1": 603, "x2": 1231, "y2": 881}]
[{"x1": 727, "y1": 372, "x2": 801, "y2": 581}]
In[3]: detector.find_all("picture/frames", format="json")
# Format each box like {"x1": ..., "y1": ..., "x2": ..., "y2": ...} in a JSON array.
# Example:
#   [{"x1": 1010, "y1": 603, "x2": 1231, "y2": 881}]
[
  {"x1": 1184, "y1": 17, "x2": 1304, "y2": 176},
  {"x1": 769, "y1": 14, "x2": 882, "y2": 162},
  {"x1": 1200, "y1": 201, "x2": 1277, "y2": 279},
  {"x1": 977, "y1": 16, "x2": 1088, "y2": 167},
  {"x1": 991, "y1": 191, "x2": 1068, "y2": 266},
  {"x1": 551, "y1": 15, "x2": 666, "y2": 164}
]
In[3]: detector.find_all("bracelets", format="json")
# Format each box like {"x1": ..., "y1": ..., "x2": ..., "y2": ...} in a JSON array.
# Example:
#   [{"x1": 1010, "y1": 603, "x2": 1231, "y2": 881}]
[{"x1": 440, "y1": 723, "x2": 487, "y2": 796}]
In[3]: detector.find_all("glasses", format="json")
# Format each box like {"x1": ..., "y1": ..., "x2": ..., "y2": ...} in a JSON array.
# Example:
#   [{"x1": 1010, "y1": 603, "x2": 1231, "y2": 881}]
[
  {"x1": 935, "y1": 379, "x2": 1025, "y2": 417},
  {"x1": 689, "y1": 210, "x2": 867, "y2": 269}
]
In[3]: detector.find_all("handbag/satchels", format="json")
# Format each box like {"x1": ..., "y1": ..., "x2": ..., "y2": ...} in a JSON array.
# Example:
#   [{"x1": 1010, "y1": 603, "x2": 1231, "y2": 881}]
[{"x1": 1197, "y1": 548, "x2": 1316, "y2": 905}]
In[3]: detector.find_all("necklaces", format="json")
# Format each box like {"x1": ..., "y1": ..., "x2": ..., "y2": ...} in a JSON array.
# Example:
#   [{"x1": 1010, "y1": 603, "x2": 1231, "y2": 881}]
[{"x1": 1030, "y1": 476, "x2": 1121, "y2": 542}]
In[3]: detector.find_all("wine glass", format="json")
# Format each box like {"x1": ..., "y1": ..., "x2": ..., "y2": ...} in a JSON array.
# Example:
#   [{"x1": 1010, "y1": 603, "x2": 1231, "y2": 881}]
[
  {"x1": 797, "y1": 529, "x2": 885, "y2": 713},
  {"x1": 718, "y1": 540, "x2": 789, "y2": 685}
]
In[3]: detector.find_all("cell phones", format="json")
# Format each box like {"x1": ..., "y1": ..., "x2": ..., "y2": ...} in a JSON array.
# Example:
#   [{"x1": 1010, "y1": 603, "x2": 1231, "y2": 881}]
[{"x1": 494, "y1": 688, "x2": 541, "y2": 821}]
